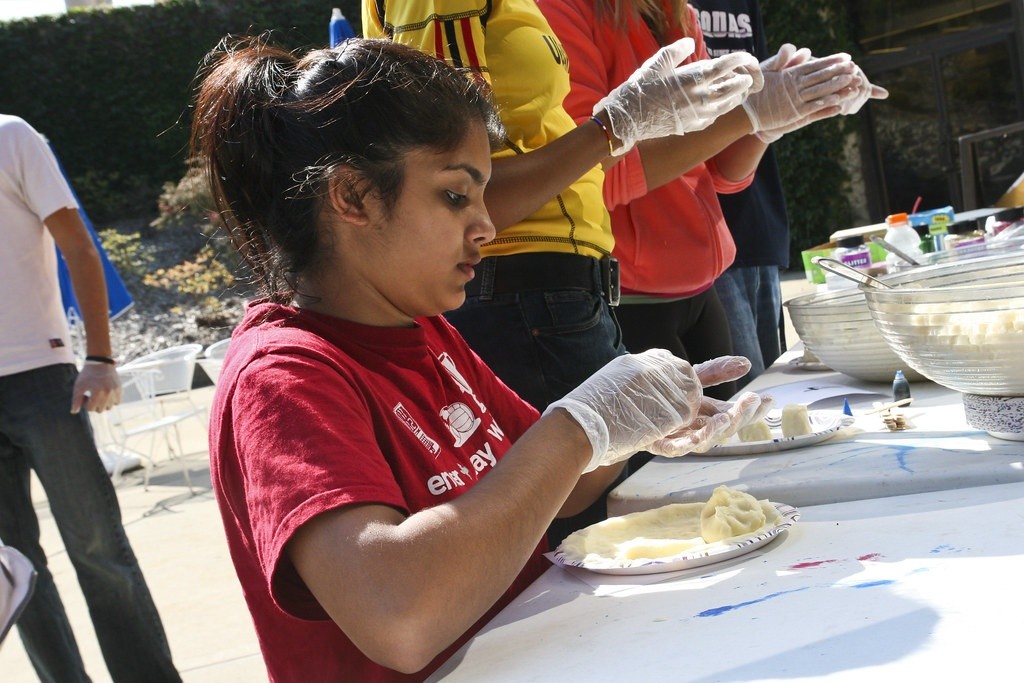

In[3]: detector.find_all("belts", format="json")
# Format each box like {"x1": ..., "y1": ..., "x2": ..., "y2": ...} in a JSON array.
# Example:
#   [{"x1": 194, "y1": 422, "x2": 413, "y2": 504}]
[{"x1": 463, "y1": 255, "x2": 620, "y2": 307}]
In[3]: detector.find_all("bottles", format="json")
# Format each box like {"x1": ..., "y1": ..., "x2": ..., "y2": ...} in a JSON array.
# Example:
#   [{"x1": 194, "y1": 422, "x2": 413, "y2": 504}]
[
  {"x1": 834, "y1": 235, "x2": 872, "y2": 270},
  {"x1": 884, "y1": 212, "x2": 923, "y2": 274}
]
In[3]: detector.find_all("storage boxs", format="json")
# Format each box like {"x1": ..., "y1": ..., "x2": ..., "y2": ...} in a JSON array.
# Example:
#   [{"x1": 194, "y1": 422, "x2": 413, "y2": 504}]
[{"x1": 803, "y1": 222, "x2": 938, "y2": 283}]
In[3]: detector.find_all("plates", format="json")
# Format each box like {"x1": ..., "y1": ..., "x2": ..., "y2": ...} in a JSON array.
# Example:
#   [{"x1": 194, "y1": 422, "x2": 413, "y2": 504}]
[
  {"x1": 553, "y1": 500, "x2": 799, "y2": 576},
  {"x1": 690, "y1": 408, "x2": 854, "y2": 456}
]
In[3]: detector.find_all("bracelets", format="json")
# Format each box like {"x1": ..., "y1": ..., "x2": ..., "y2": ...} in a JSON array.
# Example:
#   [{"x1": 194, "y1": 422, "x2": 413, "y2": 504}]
[
  {"x1": 85, "y1": 355, "x2": 119, "y2": 368},
  {"x1": 591, "y1": 117, "x2": 615, "y2": 160}
]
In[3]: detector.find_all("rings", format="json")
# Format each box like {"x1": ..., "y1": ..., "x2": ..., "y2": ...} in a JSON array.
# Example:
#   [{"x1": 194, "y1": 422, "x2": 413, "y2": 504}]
[
  {"x1": 690, "y1": 68, "x2": 703, "y2": 86},
  {"x1": 702, "y1": 91, "x2": 709, "y2": 107}
]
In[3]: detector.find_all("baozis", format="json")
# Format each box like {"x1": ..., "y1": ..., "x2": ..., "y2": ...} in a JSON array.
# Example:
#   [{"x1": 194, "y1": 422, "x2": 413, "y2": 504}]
[{"x1": 700, "y1": 485, "x2": 766, "y2": 544}]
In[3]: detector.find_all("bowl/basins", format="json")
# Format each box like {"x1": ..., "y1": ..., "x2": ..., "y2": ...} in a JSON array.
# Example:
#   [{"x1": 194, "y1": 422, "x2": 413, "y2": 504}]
[{"x1": 782, "y1": 235, "x2": 1024, "y2": 398}]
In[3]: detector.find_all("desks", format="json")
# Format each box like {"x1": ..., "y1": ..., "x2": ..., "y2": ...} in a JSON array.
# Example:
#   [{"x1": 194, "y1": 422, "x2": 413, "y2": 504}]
[
  {"x1": 607, "y1": 337, "x2": 1024, "y2": 519},
  {"x1": 419, "y1": 482, "x2": 1024, "y2": 682}
]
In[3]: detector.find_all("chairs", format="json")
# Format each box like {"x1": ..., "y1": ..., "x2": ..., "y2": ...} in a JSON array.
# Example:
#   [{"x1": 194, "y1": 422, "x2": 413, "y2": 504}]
[
  {"x1": 196, "y1": 338, "x2": 232, "y2": 385},
  {"x1": 130, "y1": 341, "x2": 202, "y2": 403},
  {"x1": 113, "y1": 343, "x2": 212, "y2": 496}
]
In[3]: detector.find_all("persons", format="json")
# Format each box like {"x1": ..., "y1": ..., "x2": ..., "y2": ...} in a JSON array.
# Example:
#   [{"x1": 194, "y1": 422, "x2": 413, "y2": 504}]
[
  {"x1": 359, "y1": 0, "x2": 890, "y2": 402},
  {"x1": 188, "y1": 39, "x2": 759, "y2": 682},
  {"x1": 0, "y1": 112, "x2": 186, "y2": 683}
]
